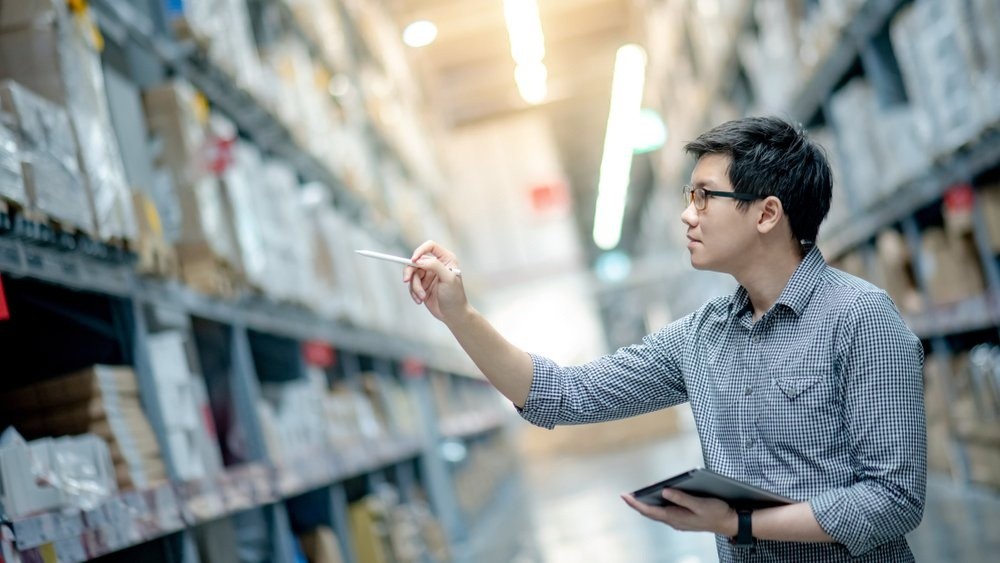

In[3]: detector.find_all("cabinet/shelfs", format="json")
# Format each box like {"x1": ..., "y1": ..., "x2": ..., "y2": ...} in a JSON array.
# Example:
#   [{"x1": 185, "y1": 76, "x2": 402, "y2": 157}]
[
  {"x1": 712, "y1": 0, "x2": 1000, "y2": 563},
  {"x1": 0, "y1": 0, "x2": 519, "y2": 563}
]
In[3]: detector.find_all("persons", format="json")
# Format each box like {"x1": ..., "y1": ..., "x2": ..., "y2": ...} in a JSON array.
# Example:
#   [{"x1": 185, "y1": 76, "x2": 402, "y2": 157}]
[{"x1": 401, "y1": 117, "x2": 927, "y2": 563}]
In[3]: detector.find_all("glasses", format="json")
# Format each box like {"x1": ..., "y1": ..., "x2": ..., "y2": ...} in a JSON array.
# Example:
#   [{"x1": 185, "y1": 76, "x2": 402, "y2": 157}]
[{"x1": 682, "y1": 185, "x2": 759, "y2": 212}]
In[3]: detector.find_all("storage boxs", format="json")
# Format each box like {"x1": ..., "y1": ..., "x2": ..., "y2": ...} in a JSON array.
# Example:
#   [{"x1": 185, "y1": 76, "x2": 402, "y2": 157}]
[
  {"x1": 0, "y1": 0, "x2": 505, "y2": 563},
  {"x1": 724, "y1": 0, "x2": 1000, "y2": 495}
]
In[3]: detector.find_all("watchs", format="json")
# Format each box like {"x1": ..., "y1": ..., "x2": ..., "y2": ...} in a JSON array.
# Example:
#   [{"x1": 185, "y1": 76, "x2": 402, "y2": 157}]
[{"x1": 727, "y1": 507, "x2": 757, "y2": 548}]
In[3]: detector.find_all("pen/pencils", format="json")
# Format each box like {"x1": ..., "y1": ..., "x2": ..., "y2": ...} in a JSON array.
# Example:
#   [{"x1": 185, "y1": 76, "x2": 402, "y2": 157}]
[{"x1": 355, "y1": 250, "x2": 461, "y2": 275}]
